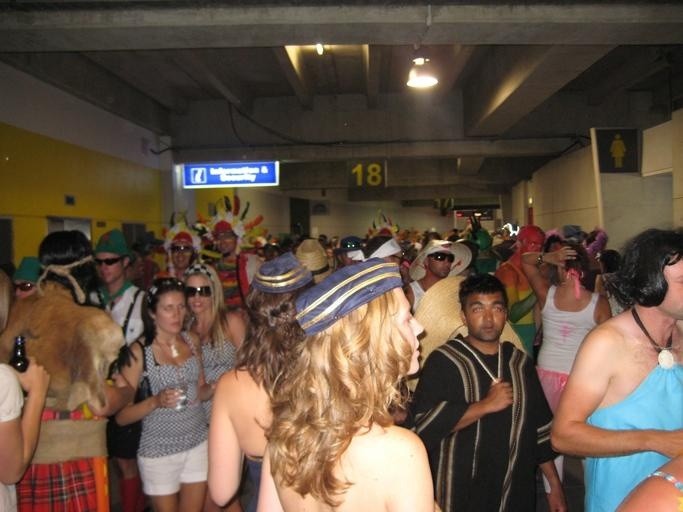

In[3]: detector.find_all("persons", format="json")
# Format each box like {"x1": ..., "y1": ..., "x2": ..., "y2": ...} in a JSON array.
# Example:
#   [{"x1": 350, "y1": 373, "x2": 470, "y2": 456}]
[{"x1": 1, "y1": 219, "x2": 683, "y2": 512}]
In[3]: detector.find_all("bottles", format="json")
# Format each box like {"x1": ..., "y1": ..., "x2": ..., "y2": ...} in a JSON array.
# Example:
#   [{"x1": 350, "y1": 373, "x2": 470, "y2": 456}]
[
  {"x1": 106, "y1": 356, "x2": 120, "y2": 387},
  {"x1": 6, "y1": 335, "x2": 29, "y2": 401}
]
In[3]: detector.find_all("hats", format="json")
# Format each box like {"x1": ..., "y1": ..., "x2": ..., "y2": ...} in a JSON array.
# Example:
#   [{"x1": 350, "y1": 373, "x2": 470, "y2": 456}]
[
  {"x1": 405, "y1": 239, "x2": 472, "y2": 284},
  {"x1": 11, "y1": 256, "x2": 43, "y2": 282},
  {"x1": 291, "y1": 257, "x2": 404, "y2": 340},
  {"x1": 91, "y1": 229, "x2": 135, "y2": 259},
  {"x1": 252, "y1": 253, "x2": 314, "y2": 297},
  {"x1": 293, "y1": 239, "x2": 333, "y2": 286},
  {"x1": 171, "y1": 230, "x2": 195, "y2": 248},
  {"x1": 515, "y1": 225, "x2": 546, "y2": 246},
  {"x1": 334, "y1": 235, "x2": 364, "y2": 254},
  {"x1": 405, "y1": 275, "x2": 525, "y2": 396}
]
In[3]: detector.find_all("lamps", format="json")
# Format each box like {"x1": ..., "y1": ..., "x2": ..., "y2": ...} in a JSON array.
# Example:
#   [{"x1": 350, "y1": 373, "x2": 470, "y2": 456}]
[{"x1": 405, "y1": 47, "x2": 441, "y2": 88}]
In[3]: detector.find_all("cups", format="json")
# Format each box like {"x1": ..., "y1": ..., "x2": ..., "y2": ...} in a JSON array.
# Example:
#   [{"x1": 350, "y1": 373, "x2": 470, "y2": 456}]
[{"x1": 168, "y1": 383, "x2": 189, "y2": 414}]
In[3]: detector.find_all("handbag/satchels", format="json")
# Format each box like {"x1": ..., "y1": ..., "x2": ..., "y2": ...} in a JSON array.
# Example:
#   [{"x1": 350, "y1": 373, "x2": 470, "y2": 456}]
[{"x1": 105, "y1": 344, "x2": 153, "y2": 459}]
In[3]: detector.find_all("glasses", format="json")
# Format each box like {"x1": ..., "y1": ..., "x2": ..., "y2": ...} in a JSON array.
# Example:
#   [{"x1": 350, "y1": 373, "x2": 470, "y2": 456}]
[
  {"x1": 95, "y1": 255, "x2": 124, "y2": 267},
  {"x1": 429, "y1": 251, "x2": 455, "y2": 267},
  {"x1": 184, "y1": 283, "x2": 213, "y2": 297},
  {"x1": 13, "y1": 282, "x2": 33, "y2": 292}
]
